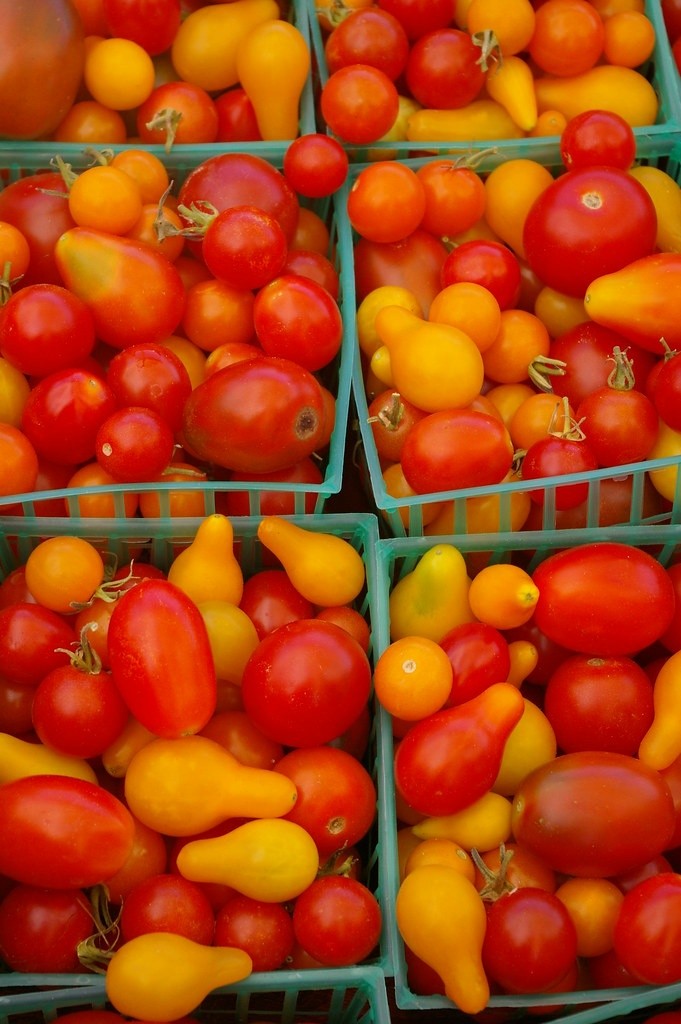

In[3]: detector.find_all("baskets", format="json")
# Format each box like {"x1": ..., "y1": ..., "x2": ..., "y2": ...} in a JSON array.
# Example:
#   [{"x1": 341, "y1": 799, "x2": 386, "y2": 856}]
[
  {"x1": 302, "y1": 0, "x2": 681, "y2": 163},
  {"x1": 336, "y1": 152, "x2": 681, "y2": 517},
  {"x1": 0, "y1": 0, "x2": 320, "y2": 184},
  {"x1": 3, "y1": 969, "x2": 393, "y2": 1024},
  {"x1": 370, "y1": 524, "x2": 681, "y2": 1012},
  {"x1": 1, "y1": 508, "x2": 395, "y2": 989},
  {"x1": 546, "y1": 985, "x2": 678, "y2": 1024},
  {"x1": 1, "y1": 136, "x2": 361, "y2": 510}
]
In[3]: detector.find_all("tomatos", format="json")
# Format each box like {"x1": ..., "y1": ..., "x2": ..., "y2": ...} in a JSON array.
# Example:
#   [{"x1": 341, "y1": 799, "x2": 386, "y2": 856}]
[{"x1": 0, "y1": 1, "x2": 681, "y2": 1024}]
